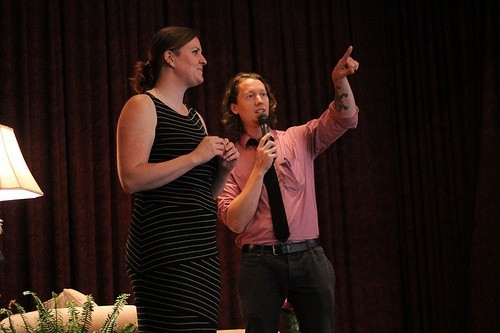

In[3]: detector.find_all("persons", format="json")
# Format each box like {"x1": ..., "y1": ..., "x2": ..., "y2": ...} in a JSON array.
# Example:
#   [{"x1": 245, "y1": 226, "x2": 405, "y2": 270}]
[
  {"x1": 115, "y1": 26, "x2": 240, "y2": 333},
  {"x1": 218, "y1": 45, "x2": 361, "y2": 333}
]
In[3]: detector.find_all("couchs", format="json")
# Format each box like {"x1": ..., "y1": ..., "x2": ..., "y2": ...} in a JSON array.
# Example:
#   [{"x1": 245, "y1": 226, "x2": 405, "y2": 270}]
[{"x1": 0, "y1": 305, "x2": 138, "y2": 333}]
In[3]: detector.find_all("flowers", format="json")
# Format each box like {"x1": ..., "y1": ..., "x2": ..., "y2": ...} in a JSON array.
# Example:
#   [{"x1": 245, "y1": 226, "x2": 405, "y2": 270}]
[{"x1": 282, "y1": 298, "x2": 300, "y2": 333}]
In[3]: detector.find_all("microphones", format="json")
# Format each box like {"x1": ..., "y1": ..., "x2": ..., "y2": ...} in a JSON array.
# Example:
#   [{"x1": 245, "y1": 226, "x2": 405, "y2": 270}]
[{"x1": 258, "y1": 113, "x2": 275, "y2": 164}]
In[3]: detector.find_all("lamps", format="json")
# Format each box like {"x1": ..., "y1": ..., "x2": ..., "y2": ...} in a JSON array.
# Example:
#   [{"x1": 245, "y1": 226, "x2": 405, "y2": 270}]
[{"x1": 0, "y1": 123, "x2": 44, "y2": 201}]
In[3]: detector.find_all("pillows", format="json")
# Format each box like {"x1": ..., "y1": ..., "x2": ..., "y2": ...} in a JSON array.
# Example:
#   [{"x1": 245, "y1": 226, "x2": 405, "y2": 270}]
[{"x1": 42, "y1": 288, "x2": 98, "y2": 309}]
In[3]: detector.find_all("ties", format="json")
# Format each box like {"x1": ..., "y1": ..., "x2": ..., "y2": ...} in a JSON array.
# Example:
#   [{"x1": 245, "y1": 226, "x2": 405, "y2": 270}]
[{"x1": 245, "y1": 136, "x2": 291, "y2": 244}]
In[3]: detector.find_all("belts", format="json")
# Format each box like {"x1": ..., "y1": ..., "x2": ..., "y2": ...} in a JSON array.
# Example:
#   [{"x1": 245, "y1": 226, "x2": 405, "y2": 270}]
[{"x1": 241, "y1": 238, "x2": 320, "y2": 256}]
